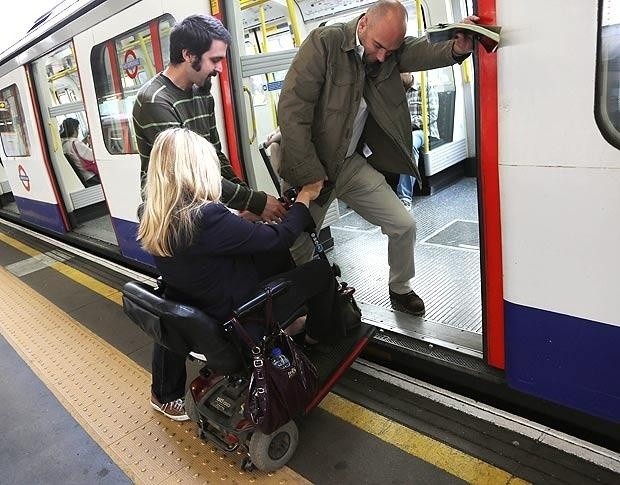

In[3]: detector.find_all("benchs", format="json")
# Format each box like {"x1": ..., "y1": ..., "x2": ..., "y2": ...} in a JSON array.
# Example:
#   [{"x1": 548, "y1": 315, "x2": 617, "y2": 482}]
[
  {"x1": 418, "y1": 88, "x2": 453, "y2": 197},
  {"x1": 64, "y1": 153, "x2": 100, "y2": 188}
]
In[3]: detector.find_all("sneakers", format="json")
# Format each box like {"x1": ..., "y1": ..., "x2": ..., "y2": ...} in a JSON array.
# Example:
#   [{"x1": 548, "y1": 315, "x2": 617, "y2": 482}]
[
  {"x1": 387, "y1": 287, "x2": 425, "y2": 316},
  {"x1": 302, "y1": 339, "x2": 334, "y2": 355},
  {"x1": 148, "y1": 385, "x2": 193, "y2": 423}
]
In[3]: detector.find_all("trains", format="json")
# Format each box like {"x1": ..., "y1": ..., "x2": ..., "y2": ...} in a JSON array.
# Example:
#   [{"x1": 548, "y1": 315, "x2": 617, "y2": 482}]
[{"x1": 0, "y1": 0, "x2": 620, "y2": 438}]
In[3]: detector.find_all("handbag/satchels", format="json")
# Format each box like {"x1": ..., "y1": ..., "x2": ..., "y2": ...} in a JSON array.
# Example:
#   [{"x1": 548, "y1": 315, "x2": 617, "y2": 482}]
[
  {"x1": 336, "y1": 282, "x2": 361, "y2": 329},
  {"x1": 72, "y1": 141, "x2": 99, "y2": 175},
  {"x1": 230, "y1": 286, "x2": 320, "y2": 434}
]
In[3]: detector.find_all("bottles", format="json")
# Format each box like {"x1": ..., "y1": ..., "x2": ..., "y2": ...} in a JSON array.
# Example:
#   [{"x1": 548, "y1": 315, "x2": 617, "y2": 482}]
[{"x1": 270, "y1": 347, "x2": 291, "y2": 371}]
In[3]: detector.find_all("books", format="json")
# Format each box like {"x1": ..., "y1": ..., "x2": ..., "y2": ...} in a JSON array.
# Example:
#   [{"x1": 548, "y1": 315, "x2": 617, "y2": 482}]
[{"x1": 423, "y1": 19, "x2": 503, "y2": 55}]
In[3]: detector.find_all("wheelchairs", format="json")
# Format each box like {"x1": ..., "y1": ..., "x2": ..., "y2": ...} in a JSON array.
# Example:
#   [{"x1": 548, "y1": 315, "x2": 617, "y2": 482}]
[{"x1": 123, "y1": 189, "x2": 377, "y2": 472}]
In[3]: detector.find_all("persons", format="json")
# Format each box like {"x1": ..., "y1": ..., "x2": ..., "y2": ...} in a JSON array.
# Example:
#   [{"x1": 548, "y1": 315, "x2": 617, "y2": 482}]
[
  {"x1": 274, "y1": 0, "x2": 481, "y2": 319},
  {"x1": 57, "y1": 116, "x2": 103, "y2": 189},
  {"x1": 396, "y1": 69, "x2": 439, "y2": 210},
  {"x1": 135, "y1": 123, "x2": 364, "y2": 351},
  {"x1": 130, "y1": 13, "x2": 291, "y2": 427}
]
